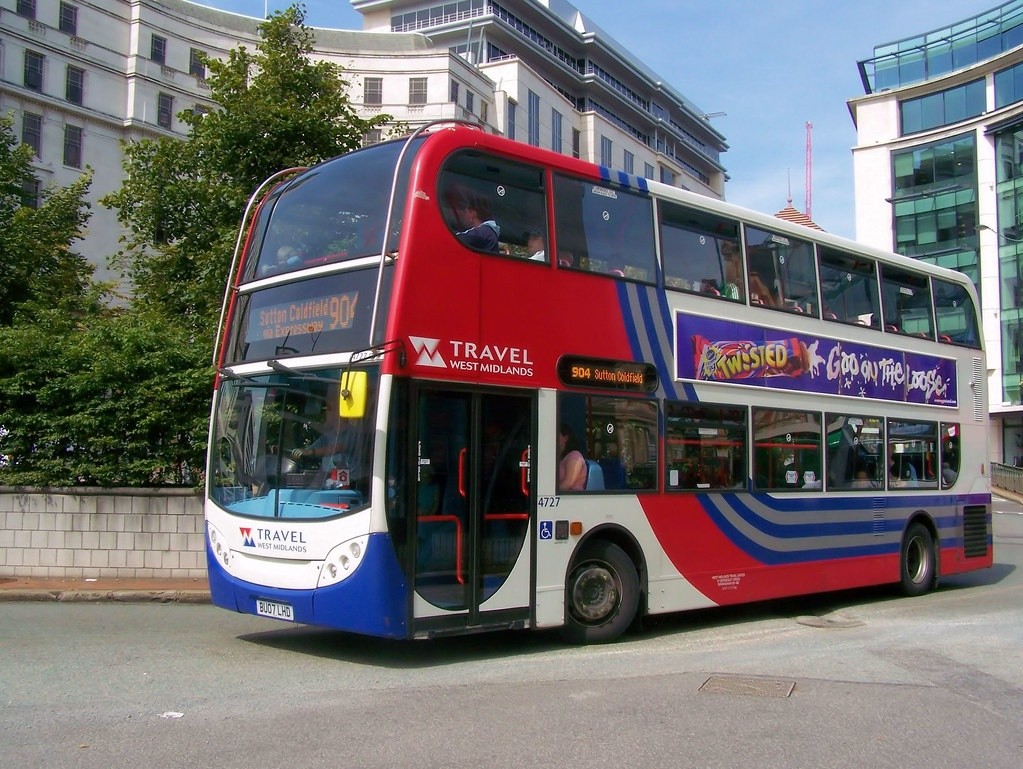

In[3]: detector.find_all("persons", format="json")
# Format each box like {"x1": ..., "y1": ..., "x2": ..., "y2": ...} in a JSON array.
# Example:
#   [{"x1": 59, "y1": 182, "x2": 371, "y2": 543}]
[
  {"x1": 558, "y1": 421, "x2": 588, "y2": 490},
  {"x1": 856, "y1": 436, "x2": 957, "y2": 488},
  {"x1": 504, "y1": 225, "x2": 545, "y2": 261},
  {"x1": 455, "y1": 196, "x2": 500, "y2": 253},
  {"x1": 268, "y1": 245, "x2": 300, "y2": 273},
  {"x1": 271, "y1": 403, "x2": 368, "y2": 483},
  {"x1": 699, "y1": 271, "x2": 835, "y2": 318}
]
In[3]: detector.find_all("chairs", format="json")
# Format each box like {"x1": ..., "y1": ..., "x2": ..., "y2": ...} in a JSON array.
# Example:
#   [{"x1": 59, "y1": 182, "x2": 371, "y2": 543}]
[
  {"x1": 849, "y1": 452, "x2": 880, "y2": 481},
  {"x1": 585, "y1": 460, "x2": 608, "y2": 492},
  {"x1": 901, "y1": 462, "x2": 919, "y2": 485}
]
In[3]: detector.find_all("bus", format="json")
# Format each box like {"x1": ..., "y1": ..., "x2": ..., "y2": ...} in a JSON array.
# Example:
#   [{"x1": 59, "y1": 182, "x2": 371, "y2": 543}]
[{"x1": 205, "y1": 120, "x2": 992, "y2": 645}]
